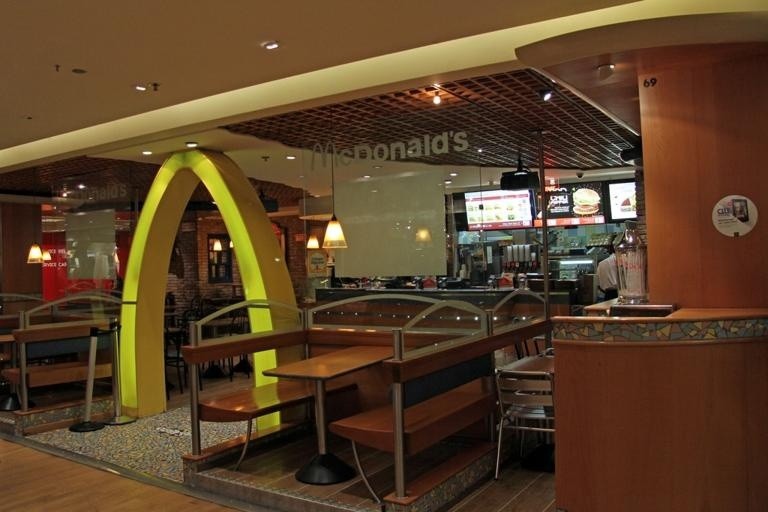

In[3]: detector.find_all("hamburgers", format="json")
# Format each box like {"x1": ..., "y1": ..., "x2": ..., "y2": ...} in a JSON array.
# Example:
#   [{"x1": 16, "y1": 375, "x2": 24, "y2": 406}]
[{"x1": 573, "y1": 187, "x2": 601, "y2": 216}]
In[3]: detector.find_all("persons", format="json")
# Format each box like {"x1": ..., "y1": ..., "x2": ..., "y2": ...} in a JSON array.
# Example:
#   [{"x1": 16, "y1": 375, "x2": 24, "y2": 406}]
[{"x1": 595, "y1": 237, "x2": 618, "y2": 303}]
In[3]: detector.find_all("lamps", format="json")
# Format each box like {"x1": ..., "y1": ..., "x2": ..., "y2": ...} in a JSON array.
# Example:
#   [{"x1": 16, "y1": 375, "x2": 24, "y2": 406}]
[
  {"x1": 213, "y1": 236, "x2": 223, "y2": 251},
  {"x1": 432, "y1": 88, "x2": 442, "y2": 105},
  {"x1": 186, "y1": 142, "x2": 198, "y2": 148},
  {"x1": 534, "y1": 88, "x2": 553, "y2": 102},
  {"x1": 596, "y1": 63, "x2": 616, "y2": 79},
  {"x1": 305, "y1": 147, "x2": 320, "y2": 250},
  {"x1": 42, "y1": 214, "x2": 51, "y2": 262},
  {"x1": 26, "y1": 167, "x2": 44, "y2": 265},
  {"x1": 320, "y1": 102, "x2": 349, "y2": 249}
]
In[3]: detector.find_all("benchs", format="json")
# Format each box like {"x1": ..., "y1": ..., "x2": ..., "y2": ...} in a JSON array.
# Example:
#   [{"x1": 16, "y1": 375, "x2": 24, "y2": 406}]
[
  {"x1": 193, "y1": 309, "x2": 554, "y2": 503},
  {"x1": 0, "y1": 319, "x2": 109, "y2": 413}
]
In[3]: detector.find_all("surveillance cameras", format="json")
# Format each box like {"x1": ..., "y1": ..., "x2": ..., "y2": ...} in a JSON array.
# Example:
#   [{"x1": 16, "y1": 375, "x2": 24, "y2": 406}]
[{"x1": 576, "y1": 173, "x2": 584, "y2": 179}]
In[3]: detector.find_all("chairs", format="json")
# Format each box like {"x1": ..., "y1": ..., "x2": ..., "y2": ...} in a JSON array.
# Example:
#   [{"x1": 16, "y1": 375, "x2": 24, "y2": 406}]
[{"x1": 166, "y1": 301, "x2": 251, "y2": 394}]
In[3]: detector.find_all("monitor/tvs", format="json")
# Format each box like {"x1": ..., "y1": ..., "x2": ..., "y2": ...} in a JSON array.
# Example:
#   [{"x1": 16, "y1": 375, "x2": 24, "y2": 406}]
[
  {"x1": 602, "y1": 178, "x2": 639, "y2": 223},
  {"x1": 463, "y1": 188, "x2": 534, "y2": 232}
]
